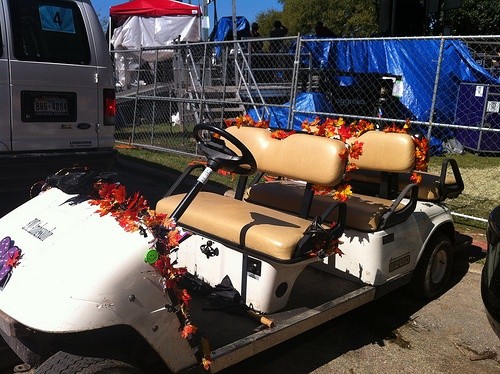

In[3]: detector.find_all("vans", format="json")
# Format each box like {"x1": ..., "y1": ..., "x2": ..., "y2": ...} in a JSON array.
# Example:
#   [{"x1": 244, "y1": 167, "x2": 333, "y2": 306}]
[{"x1": 0, "y1": 0, "x2": 116, "y2": 178}]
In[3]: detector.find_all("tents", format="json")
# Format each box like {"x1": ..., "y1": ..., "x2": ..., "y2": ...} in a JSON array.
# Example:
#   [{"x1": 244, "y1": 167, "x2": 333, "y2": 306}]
[{"x1": 106, "y1": 0, "x2": 203, "y2": 56}]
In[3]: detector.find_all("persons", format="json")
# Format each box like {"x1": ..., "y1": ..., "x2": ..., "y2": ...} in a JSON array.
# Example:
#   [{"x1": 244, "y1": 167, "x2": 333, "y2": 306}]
[
  {"x1": 314, "y1": 20, "x2": 340, "y2": 38},
  {"x1": 269, "y1": 20, "x2": 289, "y2": 54},
  {"x1": 250, "y1": 22, "x2": 264, "y2": 53}
]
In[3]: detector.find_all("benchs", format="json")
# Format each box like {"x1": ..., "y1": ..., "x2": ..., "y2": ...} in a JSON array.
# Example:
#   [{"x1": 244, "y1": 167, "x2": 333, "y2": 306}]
[
  {"x1": 156, "y1": 125, "x2": 349, "y2": 264},
  {"x1": 352, "y1": 158, "x2": 464, "y2": 202},
  {"x1": 244, "y1": 125, "x2": 421, "y2": 232}
]
